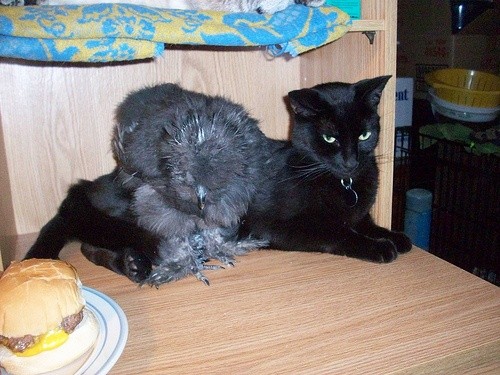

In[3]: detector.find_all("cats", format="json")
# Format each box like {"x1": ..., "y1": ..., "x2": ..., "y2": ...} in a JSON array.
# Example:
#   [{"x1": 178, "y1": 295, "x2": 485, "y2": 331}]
[{"x1": 20, "y1": 75, "x2": 413, "y2": 282}]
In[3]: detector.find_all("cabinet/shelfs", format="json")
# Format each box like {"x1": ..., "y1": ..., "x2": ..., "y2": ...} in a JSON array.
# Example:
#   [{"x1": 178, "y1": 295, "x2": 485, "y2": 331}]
[
  {"x1": 15, "y1": 233, "x2": 500, "y2": 375},
  {"x1": 0, "y1": 0, "x2": 398, "y2": 278}
]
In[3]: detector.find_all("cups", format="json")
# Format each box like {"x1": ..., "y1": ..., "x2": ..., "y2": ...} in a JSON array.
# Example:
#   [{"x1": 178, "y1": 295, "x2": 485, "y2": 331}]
[{"x1": 404, "y1": 189, "x2": 433, "y2": 251}]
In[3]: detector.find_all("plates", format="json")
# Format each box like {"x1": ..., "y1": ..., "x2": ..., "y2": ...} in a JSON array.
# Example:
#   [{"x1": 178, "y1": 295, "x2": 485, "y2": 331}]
[{"x1": 42, "y1": 285, "x2": 129, "y2": 375}]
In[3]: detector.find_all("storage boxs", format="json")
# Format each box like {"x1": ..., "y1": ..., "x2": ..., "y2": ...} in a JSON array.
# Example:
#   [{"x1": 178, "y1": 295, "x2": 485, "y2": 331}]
[{"x1": 397, "y1": 1, "x2": 500, "y2": 93}]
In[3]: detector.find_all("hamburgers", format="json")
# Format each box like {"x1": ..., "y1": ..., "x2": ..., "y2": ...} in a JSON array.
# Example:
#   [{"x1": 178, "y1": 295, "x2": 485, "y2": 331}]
[{"x1": 0, "y1": 258, "x2": 98, "y2": 375}]
